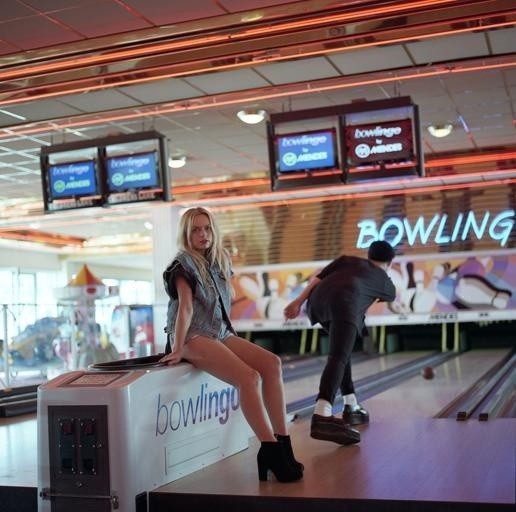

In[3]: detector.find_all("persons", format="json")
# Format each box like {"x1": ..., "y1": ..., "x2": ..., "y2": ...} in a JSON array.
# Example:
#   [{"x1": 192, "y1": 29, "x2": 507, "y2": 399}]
[
  {"x1": 162, "y1": 208, "x2": 304, "y2": 483},
  {"x1": 283, "y1": 241, "x2": 396, "y2": 444}
]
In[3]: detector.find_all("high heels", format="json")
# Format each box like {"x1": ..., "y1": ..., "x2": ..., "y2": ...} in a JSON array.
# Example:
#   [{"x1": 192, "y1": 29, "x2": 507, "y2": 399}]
[{"x1": 256, "y1": 432, "x2": 303, "y2": 484}]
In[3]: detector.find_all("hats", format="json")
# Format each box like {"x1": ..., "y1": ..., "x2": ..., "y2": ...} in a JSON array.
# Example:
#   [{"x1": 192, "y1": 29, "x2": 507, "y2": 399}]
[{"x1": 368, "y1": 239, "x2": 395, "y2": 260}]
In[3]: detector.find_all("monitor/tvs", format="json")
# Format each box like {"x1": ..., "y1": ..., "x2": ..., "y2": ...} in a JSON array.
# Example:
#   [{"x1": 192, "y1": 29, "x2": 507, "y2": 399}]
[
  {"x1": 101, "y1": 130, "x2": 170, "y2": 205},
  {"x1": 342, "y1": 96, "x2": 426, "y2": 184},
  {"x1": 40, "y1": 138, "x2": 101, "y2": 213},
  {"x1": 266, "y1": 105, "x2": 342, "y2": 192}
]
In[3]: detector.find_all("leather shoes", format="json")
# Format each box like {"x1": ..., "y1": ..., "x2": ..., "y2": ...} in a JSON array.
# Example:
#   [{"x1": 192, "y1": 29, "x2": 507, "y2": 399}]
[
  {"x1": 343, "y1": 405, "x2": 370, "y2": 425},
  {"x1": 309, "y1": 414, "x2": 361, "y2": 445}
]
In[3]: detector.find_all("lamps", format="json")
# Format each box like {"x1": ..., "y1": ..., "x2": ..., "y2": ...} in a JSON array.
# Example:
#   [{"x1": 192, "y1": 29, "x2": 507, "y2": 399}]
[
  {"x1": 236, "y1": 107, "x2": 267, "y2": 125},
  {"x1": 425, "y1": 122, "x2": 454, "y2": 138},
  {"x1": 168, "y1": 155, "x2": 187, "y2": 169}
]
